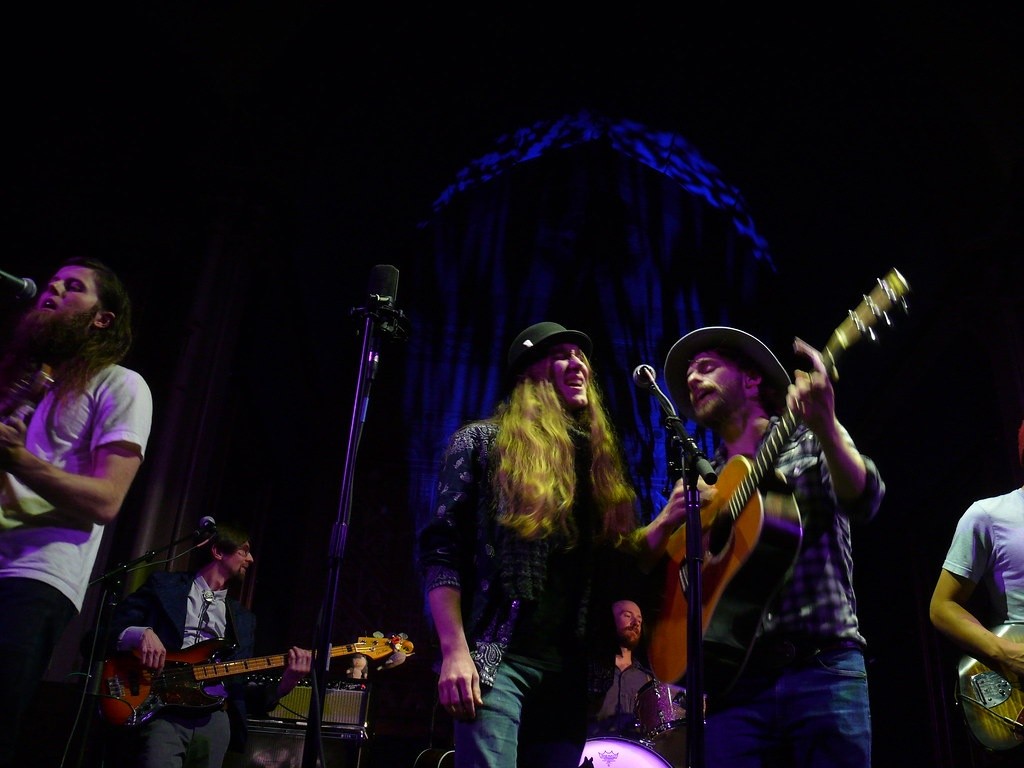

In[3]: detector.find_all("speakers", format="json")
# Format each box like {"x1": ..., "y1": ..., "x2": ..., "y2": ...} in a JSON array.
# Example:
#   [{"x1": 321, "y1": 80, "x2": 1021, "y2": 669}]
[{"x1": 221, "y1": 730, "x2": 367, "y2": 768}]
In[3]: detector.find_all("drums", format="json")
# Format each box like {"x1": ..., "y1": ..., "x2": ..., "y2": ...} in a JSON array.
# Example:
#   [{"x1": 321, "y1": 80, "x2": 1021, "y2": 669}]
[
  {"x1": 633, "y1": 686, "x2": 696, "y2": 768},
  {"x1": 573, "y1": 709, "x2": 687, "y2": 768}
]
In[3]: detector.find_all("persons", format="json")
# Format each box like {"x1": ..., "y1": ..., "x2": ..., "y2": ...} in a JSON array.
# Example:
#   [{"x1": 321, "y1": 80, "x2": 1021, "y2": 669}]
[
  {"x1": 79, "y1": 523, "x2": 310, "y2": 768},
  {"x1": 414, "y1": 322, "x2": 637, "y2": 768},
  {"x1": 929, "y1": 418, "x2": 1024, "y2": 678},
  {"x1": 590, "y1": 599, "x2": 653, "y2": 740},
  {"x1": 0, "y1": 259, "x2": 152, "y2": 734},
  {"x1": 662, "y1": 327, "x2": 886, "y2": 768}
]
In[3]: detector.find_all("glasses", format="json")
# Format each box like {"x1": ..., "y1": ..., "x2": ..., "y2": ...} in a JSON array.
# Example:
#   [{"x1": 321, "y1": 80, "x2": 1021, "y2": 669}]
[{"x1": 236, "y1": 544, "x2": 250, "y2": 554}]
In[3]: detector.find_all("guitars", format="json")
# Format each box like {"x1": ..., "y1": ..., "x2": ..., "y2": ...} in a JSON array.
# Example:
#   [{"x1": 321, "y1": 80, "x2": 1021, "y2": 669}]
[
  {"x1": 639, "y1": 268, "x2": 908, "y2": 701},
  {"x1": 948, "y1": 619, "x2": 1024, "y2": 751},
  {"x1": 0, "y1": 361, "x2": 58, "y2": 429},
  {"x1": 99, "y1": 625, "x2": 417, "y2": 729}
]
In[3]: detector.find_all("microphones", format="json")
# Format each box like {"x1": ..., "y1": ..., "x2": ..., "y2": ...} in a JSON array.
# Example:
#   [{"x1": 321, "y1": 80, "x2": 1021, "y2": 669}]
[
  {"x1": 199, "y1": 516, "x2": 217, "y2": 535},
  {"x1": 366, "y1": 265, "x2": 399, "y2": 379},
  {"x1": 0, "y1": 270, "x2": 37, "y2": 300},
  {"x1": 632, "y1": 364, "x2": 656, "y2": 389}
]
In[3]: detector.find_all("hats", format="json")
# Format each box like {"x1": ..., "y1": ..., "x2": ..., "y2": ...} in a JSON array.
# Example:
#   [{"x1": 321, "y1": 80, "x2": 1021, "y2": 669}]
[
  {"x1": 664, "y1": 326, "x2": 792, "y2": 425},
  {"x1": 506, "y1": 322, "x2": 594, "y2": 380}
]
